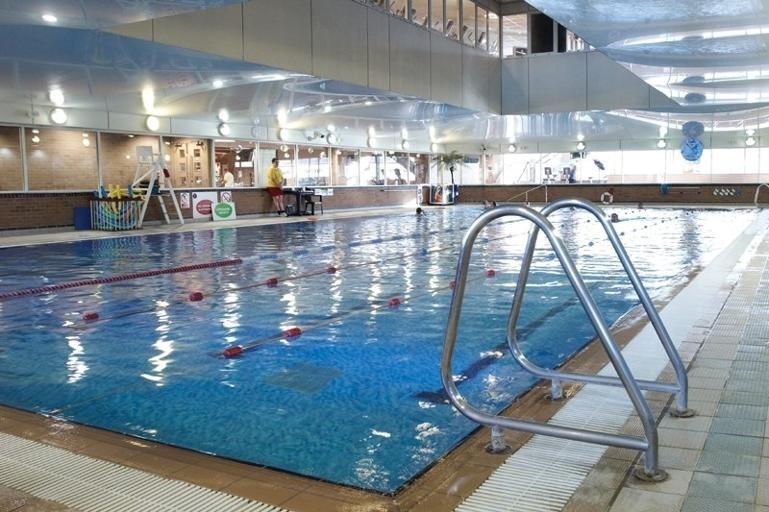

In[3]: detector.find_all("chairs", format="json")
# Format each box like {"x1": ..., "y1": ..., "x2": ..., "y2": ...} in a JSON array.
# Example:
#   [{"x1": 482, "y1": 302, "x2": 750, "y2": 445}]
[{"x1": 302, "y1": 187, "x2": 323, "y2": 214}]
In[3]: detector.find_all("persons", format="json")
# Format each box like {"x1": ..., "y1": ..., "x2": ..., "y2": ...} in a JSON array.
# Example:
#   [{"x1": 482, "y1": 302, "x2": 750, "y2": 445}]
[
  {"x1": 266, "y1": 158, "x2": 288, "y2": 217},
  {"x1": 482, "y1": 197, "x2": 496, "y2": 209},
  {"x1": 219, "y1": 168, "x2": 234, "y2": 188},
  {"x1": 415, "y1": 207, "x2": 424, "y2": 215},
  {"x1": 611, "y1": 213, "x2": 619, "y2": 222}
]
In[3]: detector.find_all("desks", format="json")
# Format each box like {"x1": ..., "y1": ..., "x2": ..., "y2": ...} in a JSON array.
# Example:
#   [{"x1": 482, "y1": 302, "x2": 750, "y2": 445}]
[{"x1": 284, "y1": 188, "x2": 314, "y2": 216}]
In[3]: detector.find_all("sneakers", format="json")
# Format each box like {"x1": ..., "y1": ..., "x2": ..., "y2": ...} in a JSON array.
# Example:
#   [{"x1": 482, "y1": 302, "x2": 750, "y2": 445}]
[{"x1": 277, "y1": 210, "x2": 287, "y2": 216}]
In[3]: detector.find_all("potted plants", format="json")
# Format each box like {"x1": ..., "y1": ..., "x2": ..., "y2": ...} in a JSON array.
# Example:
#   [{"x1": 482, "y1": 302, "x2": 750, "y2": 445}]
[{"x1": 430, "y1": 149, "x2": 465, "y2": 201}]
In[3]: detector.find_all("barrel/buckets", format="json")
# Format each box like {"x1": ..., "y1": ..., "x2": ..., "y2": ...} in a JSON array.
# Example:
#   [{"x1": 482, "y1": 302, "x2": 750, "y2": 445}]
[{"x1": 286, "y1": 204, "x2": 296, "y2": 217}]
